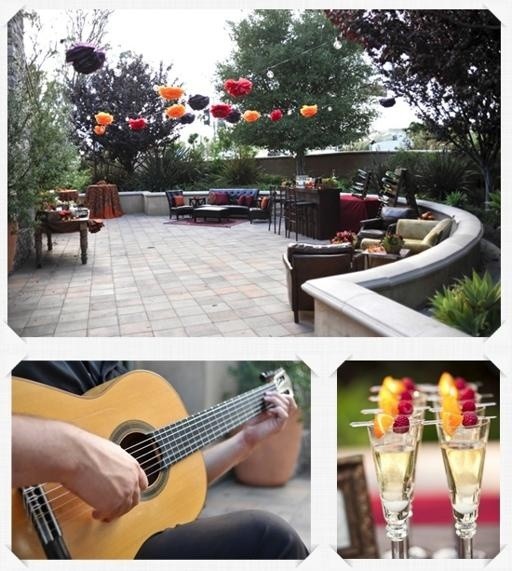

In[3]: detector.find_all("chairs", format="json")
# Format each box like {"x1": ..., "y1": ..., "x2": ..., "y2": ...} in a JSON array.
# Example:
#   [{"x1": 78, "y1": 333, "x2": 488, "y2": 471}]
[
  {"x1": 269, "y1": 185, "x2": 315, "y2": 241},
  {"x1": 166, "y1": 190, "x2": 193, "y2": 221},
  {"x1": 283, "y1": 242, "x2": 363, "y2": 323},
  {"x1": 249, "y1": 196, "x2": 274, "y2": 224}
]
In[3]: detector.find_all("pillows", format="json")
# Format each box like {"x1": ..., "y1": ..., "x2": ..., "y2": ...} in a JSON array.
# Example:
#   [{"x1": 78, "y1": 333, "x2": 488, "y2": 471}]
[
  {"x1": 209, "y1": 192, "x2": 228, "y2": 205},
  {"x1": 261, "y1": 196, "x2": 269, "y2": 210}
]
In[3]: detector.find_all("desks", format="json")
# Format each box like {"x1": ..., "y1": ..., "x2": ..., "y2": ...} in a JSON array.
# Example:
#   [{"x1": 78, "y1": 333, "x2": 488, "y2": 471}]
[
  {"x1": 284, "y1": 187, "x2": 342, "y2": 240},
  {"x1": 84, "y1": 181, "x2": 126, "y2": 219},
  {"x1": 340, "y1": 195, "x2": 380, "y2": 234},
  {"x1": 50, "y1": 190, "x2": 81, "y2": 206},
  {"x1": 33, "y1": 207, "x2": 90, "y2": 269}
]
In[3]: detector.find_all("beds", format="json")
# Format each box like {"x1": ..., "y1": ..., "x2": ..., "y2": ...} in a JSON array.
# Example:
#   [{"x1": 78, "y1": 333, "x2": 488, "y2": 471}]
[{"x1": 363, "y1": 246, "x2": 411, "y2": 270}]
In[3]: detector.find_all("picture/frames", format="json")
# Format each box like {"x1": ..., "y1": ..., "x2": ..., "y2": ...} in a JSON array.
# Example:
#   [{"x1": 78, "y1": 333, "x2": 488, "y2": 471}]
[{"x1": 336, "y1": 455, "x2": 380, "y2": 559}]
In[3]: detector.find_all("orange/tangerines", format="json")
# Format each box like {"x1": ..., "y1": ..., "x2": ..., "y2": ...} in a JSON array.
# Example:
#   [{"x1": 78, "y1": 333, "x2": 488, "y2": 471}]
[
  {"x1": 373, "y1": 375, "x2": 403, "y2": 438},
  {"x1": 438, "y1": 372, "x2": 463, "y2": 435}
]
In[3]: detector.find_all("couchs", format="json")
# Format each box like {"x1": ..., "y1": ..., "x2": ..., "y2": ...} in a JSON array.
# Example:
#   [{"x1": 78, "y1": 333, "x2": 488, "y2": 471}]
[
  {"x1": 192, "y1": 188, "x2": 258, "y2": 224},
  {"x1": 355, "y1": 207, "x2": 417, "y2": 248},
  {"x1": 360, "y1": 217, "x2": 452, "y2": 251}
]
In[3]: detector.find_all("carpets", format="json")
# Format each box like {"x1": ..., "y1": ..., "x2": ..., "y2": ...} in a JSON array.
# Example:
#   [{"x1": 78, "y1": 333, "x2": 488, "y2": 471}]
[{"x1": 164, "y1": 218, "x2": 250, "y2": 228}]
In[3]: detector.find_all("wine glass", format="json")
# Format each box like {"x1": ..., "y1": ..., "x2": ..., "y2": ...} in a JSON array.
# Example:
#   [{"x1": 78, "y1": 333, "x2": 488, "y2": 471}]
[{"x1": 367, "y1": 379, "x2": 491, "y2": 559}]
